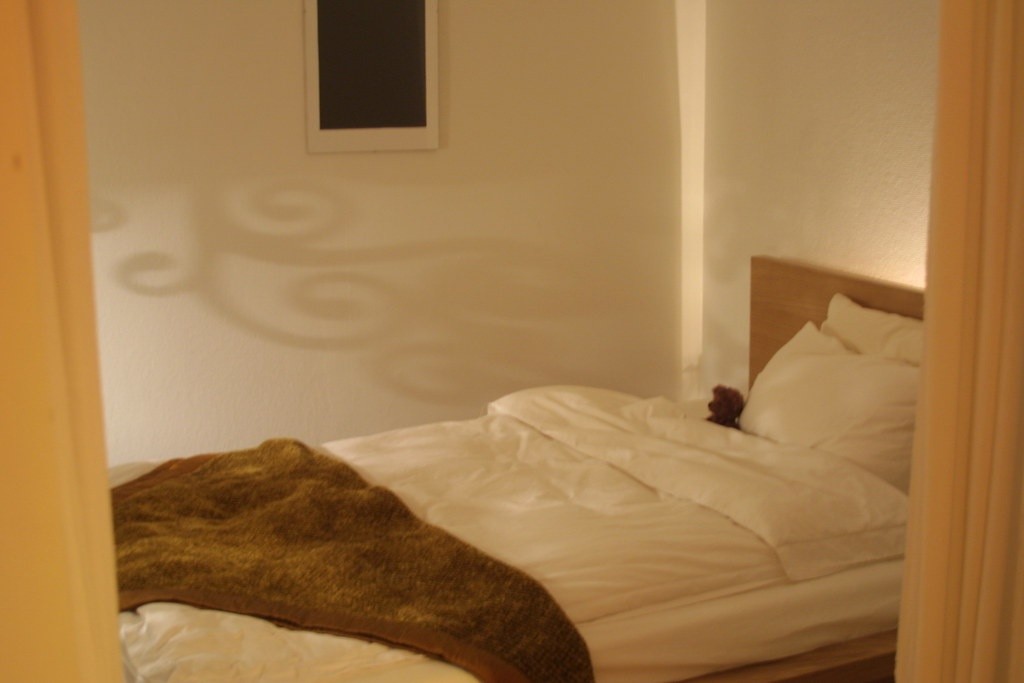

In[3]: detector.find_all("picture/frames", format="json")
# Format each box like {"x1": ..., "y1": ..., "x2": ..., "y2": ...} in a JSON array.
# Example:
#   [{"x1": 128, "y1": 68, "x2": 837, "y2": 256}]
[{"x1": 303, "y1": 0, "x2": 442, "y2": 154}]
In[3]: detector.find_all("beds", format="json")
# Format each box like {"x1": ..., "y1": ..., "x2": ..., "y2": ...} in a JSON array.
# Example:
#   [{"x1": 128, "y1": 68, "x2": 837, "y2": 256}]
[{"x1": 105, "y1": 252, "x2": 927, "y2": 683}]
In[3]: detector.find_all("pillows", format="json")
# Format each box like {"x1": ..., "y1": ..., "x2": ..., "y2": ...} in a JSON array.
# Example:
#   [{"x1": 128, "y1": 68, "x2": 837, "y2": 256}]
[
  {"x1": 734, "y1": 322, "x2": 923, "y2": 498},
  {"x1": 818, "y1": 291, "x2": 927, "y2": 366}
]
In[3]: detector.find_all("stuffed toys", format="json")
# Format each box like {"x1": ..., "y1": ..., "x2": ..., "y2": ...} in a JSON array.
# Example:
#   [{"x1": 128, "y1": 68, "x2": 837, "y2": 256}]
[{"x1": 703, "y1": 385, "x2": 744, "y2": 431}]
